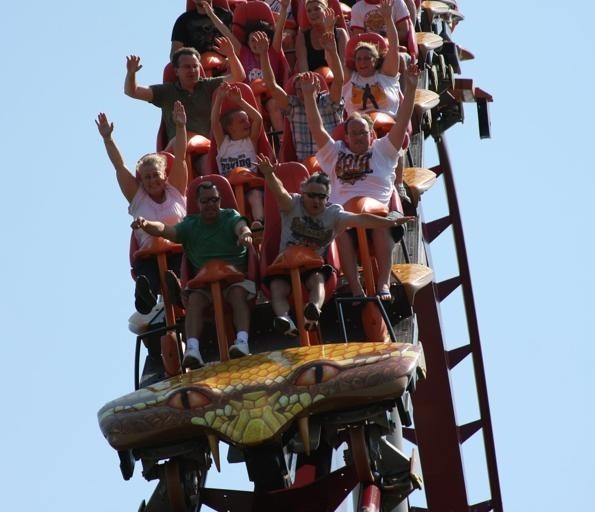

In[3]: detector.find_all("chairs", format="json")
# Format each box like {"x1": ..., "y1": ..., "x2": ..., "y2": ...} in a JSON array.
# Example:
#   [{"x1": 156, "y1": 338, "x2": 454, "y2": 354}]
[
  {"x1": 228, "y1": 338, "x2": 249, "y2": 360},
  {"x1": 273, "y1": 315, "x2": 298, "y2": 338},
  {"x1": 303, "y1": 302, "x2": 320, "y2": 332},
  {"x1": 165, "y1": 270, "x2": 181, "y2": 305},
  {"x1": 181, "y1": 349, "x2": 205, "y2": 370},
  {"x1": 134, "y1": 275, "x2": 154, "y2": 315}
]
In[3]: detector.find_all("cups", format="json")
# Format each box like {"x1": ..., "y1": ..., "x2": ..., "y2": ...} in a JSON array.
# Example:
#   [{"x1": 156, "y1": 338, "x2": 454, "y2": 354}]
[
  {"x1": 199, "y1": 196, "x2": 221, "y2": 204},
  {"x1": 302, "y1": 192, "x2": 328, "y2": 199}
]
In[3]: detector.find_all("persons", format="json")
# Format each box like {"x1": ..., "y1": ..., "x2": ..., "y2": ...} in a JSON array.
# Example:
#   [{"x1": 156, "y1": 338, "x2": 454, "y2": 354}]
[
  {"x1": 131, "y1": 179, "x2": 254, "y2": 371},
  {"x1": 94, "y1": 100, "x2": 188, "y2": 313},
  {"x1": 121, "y1": 0, "x2": 423, "y2": 302},
  {"x1": 250, "y1": 151, "x2": 416, "y2": 335}
]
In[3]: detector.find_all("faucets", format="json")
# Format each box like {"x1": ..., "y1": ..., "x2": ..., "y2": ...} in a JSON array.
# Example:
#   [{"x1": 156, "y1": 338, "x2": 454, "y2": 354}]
[
  {"x1": 351, "y1": 293, "x2": 367, "y2": 308},
  {"x1": 378, "y1": 288, "x2": 392, "y2": 302}
]
